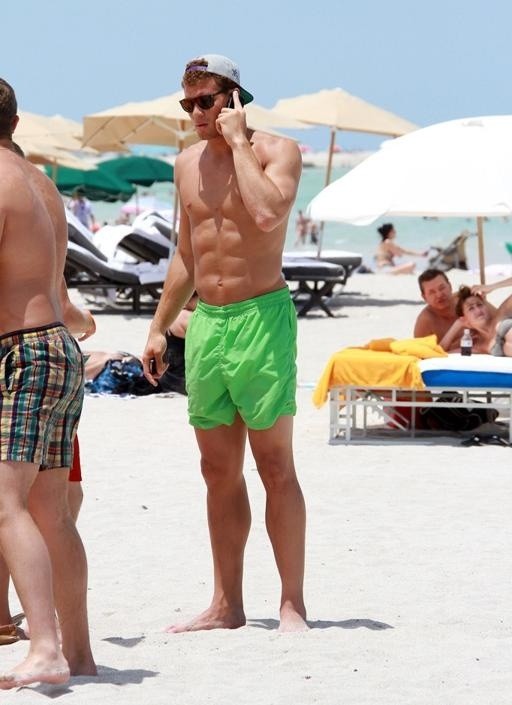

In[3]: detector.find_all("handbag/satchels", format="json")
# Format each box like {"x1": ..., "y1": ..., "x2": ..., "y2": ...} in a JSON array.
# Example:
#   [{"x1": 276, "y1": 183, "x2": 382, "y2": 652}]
[{"x1": 110, "y1": 360, "x2": 163, "y2": 394}]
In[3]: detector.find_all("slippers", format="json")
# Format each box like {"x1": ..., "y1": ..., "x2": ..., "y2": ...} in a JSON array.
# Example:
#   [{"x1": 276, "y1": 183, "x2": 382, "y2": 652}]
[{"x1": 461, "y1": 435, "x2": 512, "y2": 447}]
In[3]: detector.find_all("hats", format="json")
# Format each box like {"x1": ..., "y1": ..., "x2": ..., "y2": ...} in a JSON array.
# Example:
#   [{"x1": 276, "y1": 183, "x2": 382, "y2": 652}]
[{"x1": 184, "y1": 54, "x2": 253, "y2": 105}]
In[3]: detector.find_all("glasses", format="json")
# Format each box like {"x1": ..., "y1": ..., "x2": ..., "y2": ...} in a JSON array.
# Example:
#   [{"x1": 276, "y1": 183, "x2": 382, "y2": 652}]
[{"x1": 179, "y1": 89, "x2": 226, "y2": 113}]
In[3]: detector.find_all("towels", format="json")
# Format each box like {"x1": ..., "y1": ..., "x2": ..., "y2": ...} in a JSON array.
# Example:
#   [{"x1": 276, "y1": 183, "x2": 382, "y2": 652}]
[{"x1": 313, "y1": 332, "x2": 446, "y2": 413}]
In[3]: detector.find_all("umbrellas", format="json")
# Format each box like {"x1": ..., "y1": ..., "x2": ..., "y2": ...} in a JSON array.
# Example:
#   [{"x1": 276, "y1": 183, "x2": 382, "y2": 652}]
[{"x1": 299, "y1": 112, "x2": 512, "y2": 303}]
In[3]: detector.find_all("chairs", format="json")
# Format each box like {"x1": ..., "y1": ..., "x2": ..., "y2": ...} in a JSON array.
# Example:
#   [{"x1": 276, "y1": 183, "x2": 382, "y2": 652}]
[
  {"x1": 138, "y1": 210, "x2": 362, "y2": 298},
  {"x1": 93, "y1": 226, "x2": 347, "y2": 318},
  {"x1": 59, "y1": 208, "x2": 198, "y2": 314},
  {"x1": 325, "y1": 344, "x2": 511, "y2": 444}
]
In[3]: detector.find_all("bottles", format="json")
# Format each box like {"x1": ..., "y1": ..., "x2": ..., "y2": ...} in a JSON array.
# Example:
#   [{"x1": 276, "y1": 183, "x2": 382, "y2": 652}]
[{"x1": 459, "y1": 327, "x2": 473, "y2": 356}]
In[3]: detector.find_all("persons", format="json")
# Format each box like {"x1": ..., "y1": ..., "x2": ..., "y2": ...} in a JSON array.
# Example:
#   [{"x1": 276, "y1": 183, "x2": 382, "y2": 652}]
[
  {"x1": 413, "y1": 268, "x2": 498, "y2": 355},
  {"x1": 137, "y1": 51, "x2": 309, "y2": 640},
  {"x1": 438, "y1": 275, "x2": 512, "y2": 356},
  {"x1": 1, "y1": 141, "x2": 83, "y2": 650},
  {"x1": 0, "y1": 75, "x2": 99, "y2": 693}
]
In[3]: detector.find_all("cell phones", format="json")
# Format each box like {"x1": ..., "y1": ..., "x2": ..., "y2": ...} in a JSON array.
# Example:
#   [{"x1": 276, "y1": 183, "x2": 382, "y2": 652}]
[{"x1": 227, "y1": 94, "x2": 245, "y2": 109}]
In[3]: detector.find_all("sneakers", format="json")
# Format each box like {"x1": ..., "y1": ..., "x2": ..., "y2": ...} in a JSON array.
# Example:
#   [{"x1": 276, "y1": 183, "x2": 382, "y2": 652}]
[{"x1": 419, "y1": 392, "x2": 499, "y2": 431}]
